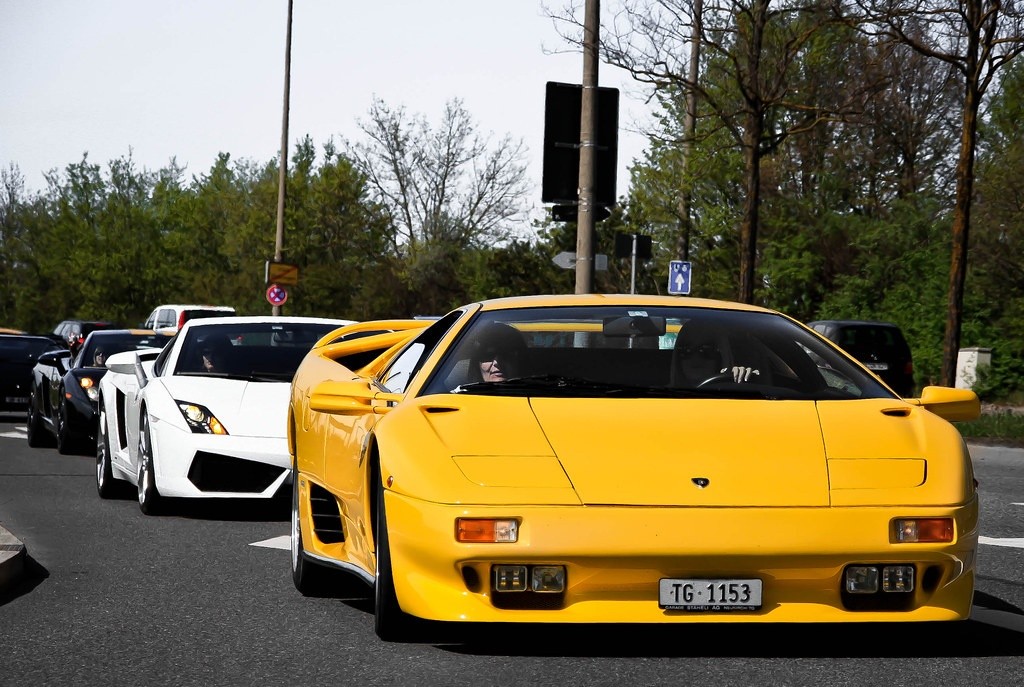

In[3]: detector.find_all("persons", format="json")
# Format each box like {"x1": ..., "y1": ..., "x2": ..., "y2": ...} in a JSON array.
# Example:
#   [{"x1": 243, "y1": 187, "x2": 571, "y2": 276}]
[
  {"x1": 201, "y1": 334, "x2": 235, "y2": 372},
  {"x1": 666, "y1": 318, "x2": 760, "y2": 389},
  {"x1": 93, "y1": 346, "x2": 111, "y2": 367},
  {"x1": 449, "y1": 323, "x2": 536, "y2": 393}
]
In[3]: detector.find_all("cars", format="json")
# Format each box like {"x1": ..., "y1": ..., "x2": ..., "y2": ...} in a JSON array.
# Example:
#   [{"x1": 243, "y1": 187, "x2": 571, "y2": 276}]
[{"x1": 0, "y1": 305, "x2": 235, "y2": 456}]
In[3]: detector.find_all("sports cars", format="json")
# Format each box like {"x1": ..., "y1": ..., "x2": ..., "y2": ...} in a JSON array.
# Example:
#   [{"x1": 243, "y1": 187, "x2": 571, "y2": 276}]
[
  {"x1": 281, "y1": 290, "x2": 982, "y2": 641},
  {"x1": 97, "y1": 316, "x2": 428, "y2": 515}
]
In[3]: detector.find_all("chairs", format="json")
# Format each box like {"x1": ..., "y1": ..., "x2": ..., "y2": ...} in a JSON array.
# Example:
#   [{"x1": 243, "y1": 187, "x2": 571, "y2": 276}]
[
  {"x1": 444, "y1": 342, "x2": 811, "y2": 400},
  {"x1": 194, "y1": 343, "x2": 307, "y2": 377}
]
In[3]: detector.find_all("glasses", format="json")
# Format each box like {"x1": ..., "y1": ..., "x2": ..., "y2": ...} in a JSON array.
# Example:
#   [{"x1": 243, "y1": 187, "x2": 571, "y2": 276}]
[
  {"x1": 200, "y1": 346, "x2": 224, "y2": 356},
  {"x1": 675, "y1": 343, "x2": 720, "y2": 361},
  {"x1": 480, "y1": 349, "x2": 518, "y2": 362},
  {"x1": 94, "y1": 351, "x2": 109, "y2": 356}
]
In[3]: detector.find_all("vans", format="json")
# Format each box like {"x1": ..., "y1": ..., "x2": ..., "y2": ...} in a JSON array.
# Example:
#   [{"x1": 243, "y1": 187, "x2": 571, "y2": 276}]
[{"x1": 800, "y1": 318, "x2": 916, "y2": 402}]
